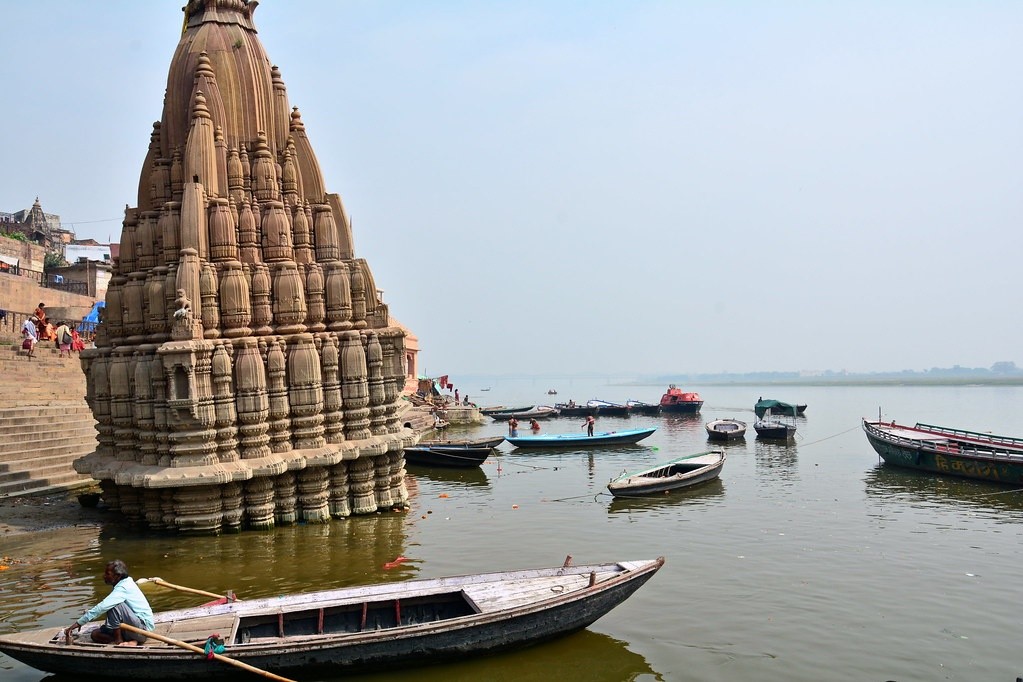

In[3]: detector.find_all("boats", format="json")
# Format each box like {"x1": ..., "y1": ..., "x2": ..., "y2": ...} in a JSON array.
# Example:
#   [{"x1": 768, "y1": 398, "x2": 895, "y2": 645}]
[
  {"x1": 586, "y1": 399, "x2": 624, "y2": 408},
  {"x1": 503, "y1": 428, "x2": 657, "y2": 448},
  {"x1": 480, "y1": 398, "x2": 562, "y2": 420},
  {"x1": 753, "y1": 396, "x2": 807, "y2": 416},
  {"x1": 553, "y1": 403, "x2": 631, "y2": 416},
  {"x1": 402, "y1": 445, "x2": 495, "y2": 468},
  {"x1": 1, "y1": 557, "x2": 666, "y2": 681},
  {"x1": 660, "y1": 384, "x2": 705, "y2": 412},
  {"x1": 859, "y1": 406, "x2": 1022, "y2": 480},
  {"x1": 704, "y1": 417, "x2": 748, "y2": 439},
  {"x1": 414, "y1": 436, "x2": 506, "y2": 451},
  {"x1": 605, "y1": 448, "x2": 726, "y2": 498},
  {"x1": 753, "y1": 418, "x2": 796, "y2": 440},
  {"x1": 626, "y1": 400, "x2": 662, "y2": 414}
]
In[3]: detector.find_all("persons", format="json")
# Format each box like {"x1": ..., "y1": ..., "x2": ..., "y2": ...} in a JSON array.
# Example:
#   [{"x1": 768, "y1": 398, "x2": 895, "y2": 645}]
[
  {"x1": 586, "y1": 412, "x2": 594, "y2": 436},
  {"x1": 530, "y1": 419, "x2": 540, "y2": 429},
  {"x1": 22, "y1": 303, "x2": 85, "y2": 357},
  {"x1": 509, "y1": 416, "x2": 518, "y2": 437},
  {"x1": 454, "y1": 388, "x2": 459, "y2": 405},
  {"x1": 464, "y1": 395, "x2": 468, "y2": 402},
  {"x1": 64, "y1": 560, "x2": 155, "y2": 646}
]
